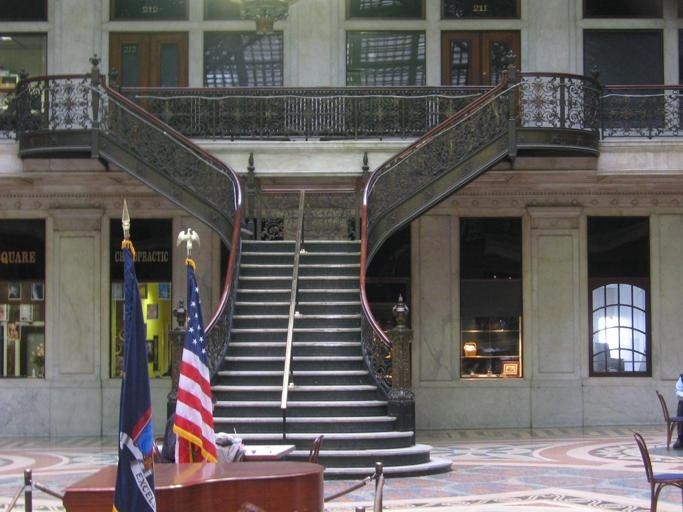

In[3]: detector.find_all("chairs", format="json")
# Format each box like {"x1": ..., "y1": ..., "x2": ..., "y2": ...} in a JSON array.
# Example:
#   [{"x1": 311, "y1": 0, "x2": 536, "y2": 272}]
[
  {"x1": 656, "y1": 389, "x2": 683, "y2": 449},
  {"x1": 633, "y1": 431, "x2": 683, "y2": 512}
]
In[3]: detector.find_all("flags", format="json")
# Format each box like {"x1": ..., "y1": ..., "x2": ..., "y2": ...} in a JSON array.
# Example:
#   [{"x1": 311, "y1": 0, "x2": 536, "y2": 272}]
[
  {"x1": 112, "y1": 237, "x2": 156, "y2": 511},
  {"x1": 171, "y1": 258, "x2": 215, "y2": 463}
]
